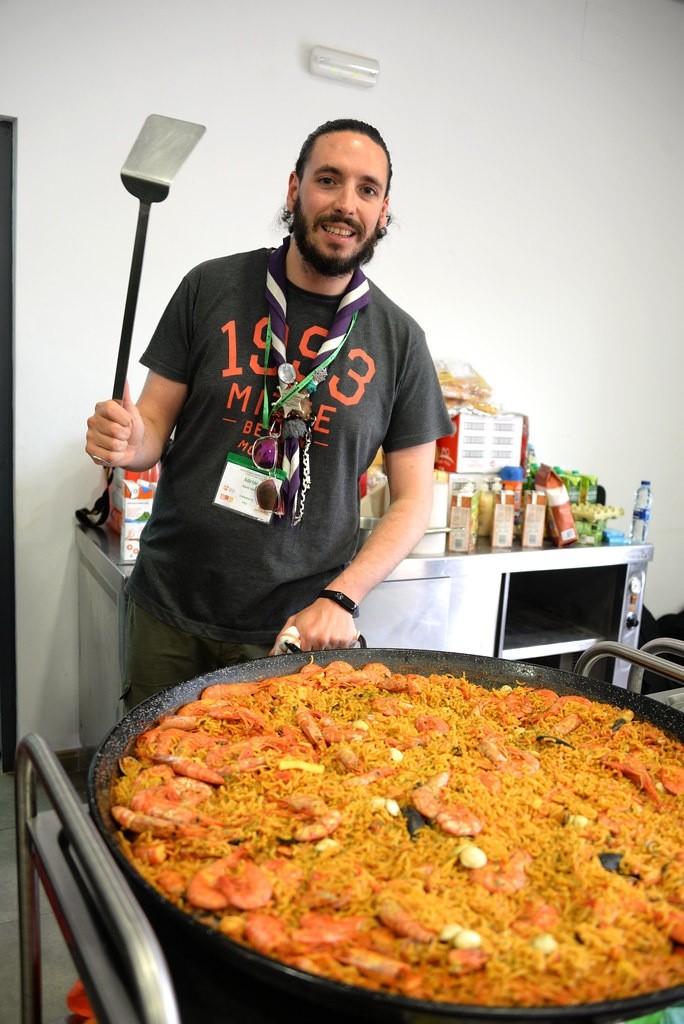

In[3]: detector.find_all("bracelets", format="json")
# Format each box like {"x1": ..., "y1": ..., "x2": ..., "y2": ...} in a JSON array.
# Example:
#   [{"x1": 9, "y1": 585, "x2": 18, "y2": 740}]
[{"x1": 318, "y1": 589, "x2": 359, "y2": 617}]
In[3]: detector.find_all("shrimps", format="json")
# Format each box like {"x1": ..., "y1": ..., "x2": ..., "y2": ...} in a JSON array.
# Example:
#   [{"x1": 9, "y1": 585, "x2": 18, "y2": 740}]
[{"x1": 107, "y1": 657, "x2": 684, "y2": 998}]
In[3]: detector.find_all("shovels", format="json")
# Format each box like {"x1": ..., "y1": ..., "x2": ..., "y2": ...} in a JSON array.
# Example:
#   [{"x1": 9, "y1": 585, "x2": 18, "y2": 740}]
[{"x1": 113, "y1": 114, "x2": 257, "y2": 398}]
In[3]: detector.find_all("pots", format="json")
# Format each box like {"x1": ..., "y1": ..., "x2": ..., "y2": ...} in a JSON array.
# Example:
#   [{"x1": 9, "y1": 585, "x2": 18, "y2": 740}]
[{"x1": 88, "y1": 649, "x2": 683, "y2": 1016}]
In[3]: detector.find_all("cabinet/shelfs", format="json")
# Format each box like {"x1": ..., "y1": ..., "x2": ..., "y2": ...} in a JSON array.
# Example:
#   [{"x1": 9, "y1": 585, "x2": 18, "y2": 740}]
[{"x1": 75, "y1": 525, "x2": 654, "y2": 741}]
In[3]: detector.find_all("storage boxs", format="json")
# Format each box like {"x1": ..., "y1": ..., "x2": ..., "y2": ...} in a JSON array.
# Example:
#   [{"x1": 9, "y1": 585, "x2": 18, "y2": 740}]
[
  {"x1": 111, "y1": 490, "x2": 154, "y2": 565},
  {"x1": 435, "y1": 469, "x2": 523, "y2": 536},
  {"x1": 436, "y1": 413, "x2": 524, "y2": 473}
]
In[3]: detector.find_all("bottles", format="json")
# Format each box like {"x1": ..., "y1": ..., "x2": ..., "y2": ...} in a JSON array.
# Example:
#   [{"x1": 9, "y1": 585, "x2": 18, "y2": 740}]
[{"x1": 630, "y1": 481, "x2": 653, "y2": 543}]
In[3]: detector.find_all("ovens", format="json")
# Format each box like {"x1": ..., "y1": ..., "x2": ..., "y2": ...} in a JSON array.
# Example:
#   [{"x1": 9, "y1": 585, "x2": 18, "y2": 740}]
[{"x1": 494, "y1": 547, "x2": 654, "y2": 690}]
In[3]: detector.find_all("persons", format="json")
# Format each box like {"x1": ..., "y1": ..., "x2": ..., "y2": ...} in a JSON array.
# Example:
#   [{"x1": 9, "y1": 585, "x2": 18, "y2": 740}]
[{"x1": 86, "y1": 119, "x2": 455, "y2": 713}]
